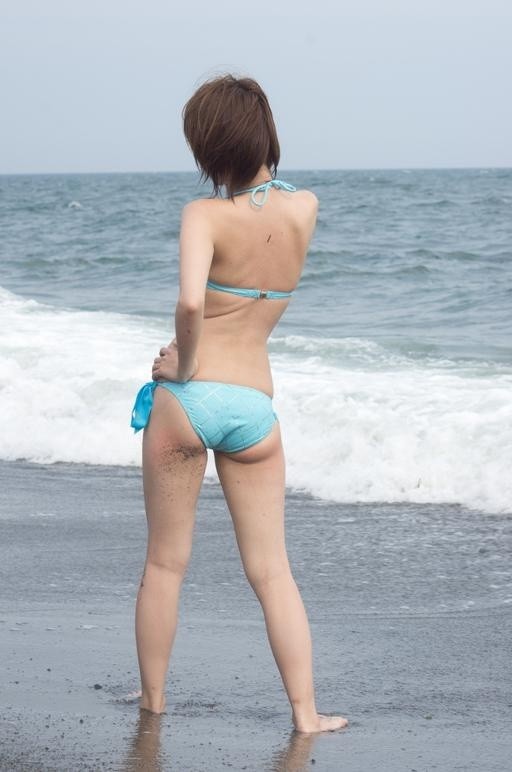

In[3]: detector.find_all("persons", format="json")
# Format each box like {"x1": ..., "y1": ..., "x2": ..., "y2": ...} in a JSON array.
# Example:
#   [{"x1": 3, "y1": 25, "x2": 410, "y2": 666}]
[{"x1": 130, "y1": 73, "x2": 348, "y2": 734}]
